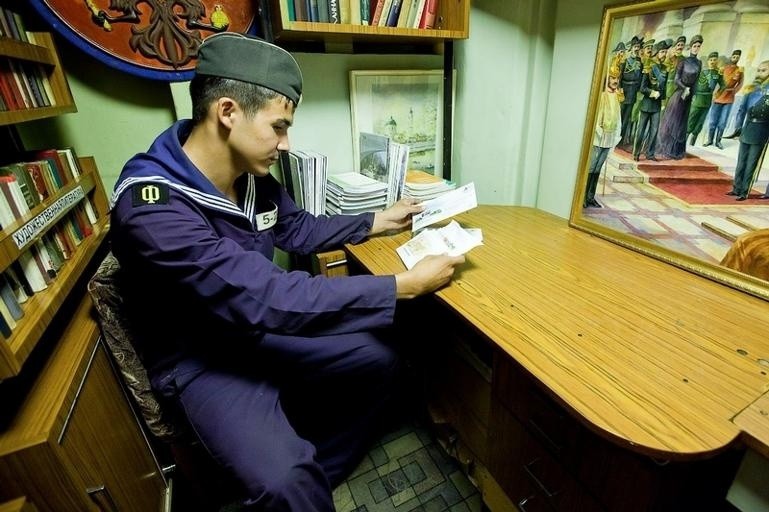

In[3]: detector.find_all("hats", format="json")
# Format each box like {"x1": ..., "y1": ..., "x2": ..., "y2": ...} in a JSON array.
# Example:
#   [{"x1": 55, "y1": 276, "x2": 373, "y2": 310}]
[
  {"x1": 626, "y1": 35, "x2": 673, "y2": 57},
  {"x1": 612, "y1": 42, "x2": 627, "y2": 53},
  {"x1": 195, "y1": 31, "x2": 306, "y2": 110},
  {"x1": 689, "y1": 34, "x2": 703, "y2": 47},
  {"x1": 674, "y1": 36, "x2": 686, "y2": 46},
  {"x1": 707, "y1": 52, "x2": 718, "y2": 60},
  {"x1": 732, "y1": 50, "x2": 741, "y2": 56}
]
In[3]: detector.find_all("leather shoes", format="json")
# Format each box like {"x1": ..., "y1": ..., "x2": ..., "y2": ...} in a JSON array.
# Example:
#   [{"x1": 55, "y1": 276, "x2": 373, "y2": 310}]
[
  {"x1": 646, "y1": 155, "x2": 658, "y2": 162},
  {"x1": 735, "y1": 193, "x2": 746, "y2": 201},
  {"x1": 633, "y1": 154, "x2": 639, "y2": 162},
  {"x1": 721, "y1": 128, "x2": 742, "y2": 139},
  {"x1": 725, "y1": 189, "x2": 739, "y2": 196},
  {"x1": 756, "y1": 193, "x2": 769, "y2": 200}
]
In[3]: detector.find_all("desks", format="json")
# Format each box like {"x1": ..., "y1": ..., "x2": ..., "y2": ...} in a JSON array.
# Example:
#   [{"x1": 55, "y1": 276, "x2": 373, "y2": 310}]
[{"x1": 342, "y1": 203, "x2": 769, "y2": 461}]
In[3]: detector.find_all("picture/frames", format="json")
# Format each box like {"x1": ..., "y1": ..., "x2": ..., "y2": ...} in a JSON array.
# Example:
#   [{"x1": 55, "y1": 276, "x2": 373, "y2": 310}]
[
  {"x1": 348, "y1": 69, "x2": 457, "y2": 182},
  {"x1": 567, "y1": 0, "x2": 769, "y2": 300}
]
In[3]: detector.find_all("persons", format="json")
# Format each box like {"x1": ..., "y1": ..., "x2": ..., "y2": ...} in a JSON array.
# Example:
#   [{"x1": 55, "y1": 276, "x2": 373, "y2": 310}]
[
  {"x1": 581, "y1": 31, "x2": 769, "y2": 211},
  {"x1": 106, "y1": 30, "x2": 469, "y2": 512}
]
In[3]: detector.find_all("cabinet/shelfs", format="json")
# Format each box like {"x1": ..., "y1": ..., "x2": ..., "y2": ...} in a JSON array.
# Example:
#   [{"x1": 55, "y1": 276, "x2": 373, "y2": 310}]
[
  {"x1": 266, "y1": 0, "x2": 470, "y2": 40},
  {"x1": 0, "y1": 27, "x2": 115, "y2": 379},
  {"x1": 0, "y1": 287, "x2": 173, "y2": 512}
]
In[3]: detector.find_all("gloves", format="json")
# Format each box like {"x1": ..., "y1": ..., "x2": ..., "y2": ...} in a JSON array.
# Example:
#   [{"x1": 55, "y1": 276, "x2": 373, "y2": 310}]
[{"x1": 650, "y1": 90, "x2": 661, "y2": 100}]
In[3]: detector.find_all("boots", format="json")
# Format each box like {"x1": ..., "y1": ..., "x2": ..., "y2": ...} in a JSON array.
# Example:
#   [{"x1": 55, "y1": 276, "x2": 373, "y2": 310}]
[
  {"x1": 716, "y1": 128, "x2": 724, "y2": 150},
  {"x1": 689, "y1": 134, "x2": 697, "y2": 146},
  {"x1": 703, "y1": 127, "x2": 715, "y2": 147},
  {"x1": 583, "y1": 173, "x2": 601, "y2": 209}
]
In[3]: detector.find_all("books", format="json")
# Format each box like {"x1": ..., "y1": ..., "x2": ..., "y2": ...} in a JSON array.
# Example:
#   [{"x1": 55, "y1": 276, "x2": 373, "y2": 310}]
[
  {"x1": 285, "y1": 1, "x2": 438, "y2": 31},
  {"x1": 278, "y1": 131, "x2": 459, "y2": 219},
  {"x1": 1, "y1": 2, "x2": 99, "y2": 348}
]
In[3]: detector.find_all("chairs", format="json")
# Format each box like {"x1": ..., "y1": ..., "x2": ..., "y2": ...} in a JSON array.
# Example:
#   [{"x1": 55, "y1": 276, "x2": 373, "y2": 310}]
[{"x1": 87, "y1": 250, "x2": 361, "y2": 512}]
